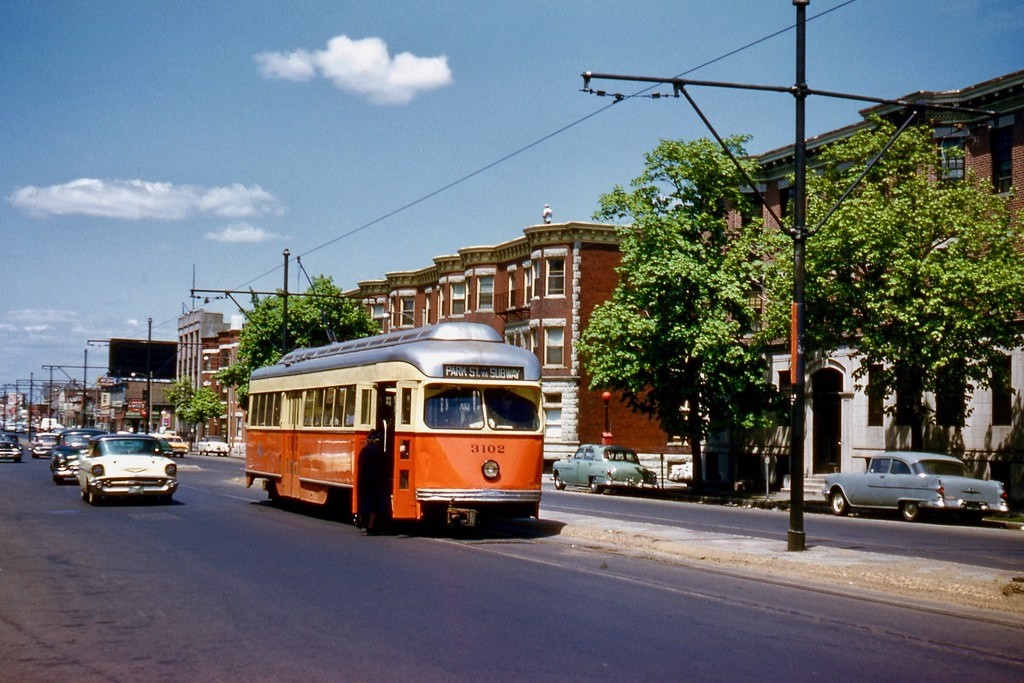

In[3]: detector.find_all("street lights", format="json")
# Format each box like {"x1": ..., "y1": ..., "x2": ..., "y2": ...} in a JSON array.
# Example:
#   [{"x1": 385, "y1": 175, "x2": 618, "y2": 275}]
[{"x1": 602, "y1": 390, "x2": 612, "y2": 447}]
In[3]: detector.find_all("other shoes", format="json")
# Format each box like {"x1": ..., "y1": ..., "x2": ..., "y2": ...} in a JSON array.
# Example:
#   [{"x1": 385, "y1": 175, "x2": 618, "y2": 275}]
[
  {"x1": 366, "y1": 527, "x2": 384, "y2": 535},
  {"x1": 362, "y1": 520, "x2": 377, "y2": 532}
]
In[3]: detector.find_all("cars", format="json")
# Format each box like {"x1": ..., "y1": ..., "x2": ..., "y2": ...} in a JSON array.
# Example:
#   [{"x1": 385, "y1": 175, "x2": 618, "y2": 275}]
[
  {"x1": 150, "y1": 434, "x2": 189, "y2": 458},
  {"x1": 670, "y1": 460, "x2": 695, "y2": 488},
  {"x1": 824, "y1": 453, "x2": 1008, "y2": 523},
  {"x1": 28, "y1": 433, "x2": 60, "y2": 459},
  {"x1": 0, "y1": 417, "x2": 75, "y2": 435},
  {"x1": 0, "y1": 434, "x2": 24, "y2": 463},
  {"x1": 198, "y1": 435, "x2": 231, "y2": 459},
  {"x1": 78, "y1": 434, "x2": 180, "y2": 506},
  {"x1": 50, "y1": 429, "x2": 110, "y2": 485},
  {"x1": 554, "y1": 444, "x2": 658, "y2": 495}
]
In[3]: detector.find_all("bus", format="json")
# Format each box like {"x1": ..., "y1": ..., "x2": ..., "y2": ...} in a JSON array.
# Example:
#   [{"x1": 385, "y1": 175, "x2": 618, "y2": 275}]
[{"x1": 245, "y1": 321, "x2": 548, "y2": 531}]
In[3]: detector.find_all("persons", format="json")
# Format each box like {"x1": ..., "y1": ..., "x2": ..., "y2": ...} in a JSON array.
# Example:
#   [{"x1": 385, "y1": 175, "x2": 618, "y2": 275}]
[
  {"x1": 543, "y1": 205, "x2": 552, "y2": 224},
  {"x1": 358, "y1": 429, "x2": 392, "y2": 536}
]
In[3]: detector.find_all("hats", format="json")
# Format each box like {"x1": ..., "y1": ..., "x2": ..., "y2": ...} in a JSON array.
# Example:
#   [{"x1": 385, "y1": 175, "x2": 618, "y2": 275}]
[{"x1": 368, "y1": 429, "x2": 380, "y2": 439}]
